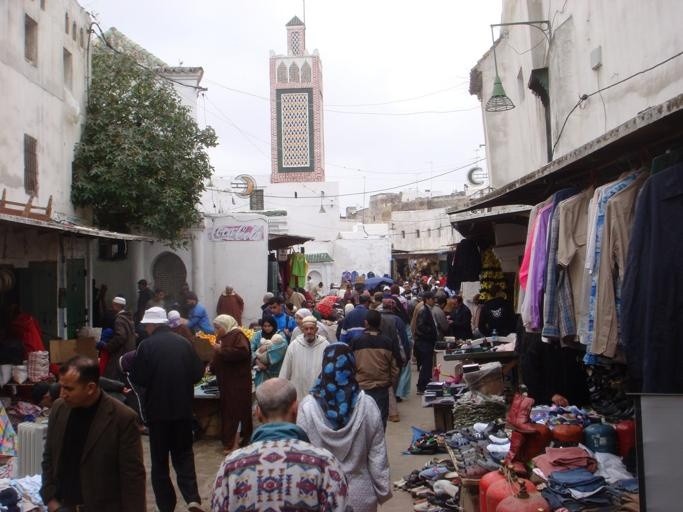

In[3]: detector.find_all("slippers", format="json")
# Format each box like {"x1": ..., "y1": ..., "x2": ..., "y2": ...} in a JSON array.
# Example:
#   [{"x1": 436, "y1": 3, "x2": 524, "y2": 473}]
[{"x1": 444, "y1": 418, "x2": 510, "y2": 478}]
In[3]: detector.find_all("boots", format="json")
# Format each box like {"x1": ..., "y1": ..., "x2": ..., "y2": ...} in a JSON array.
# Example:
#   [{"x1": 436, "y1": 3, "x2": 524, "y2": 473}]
[
  {"x1": 507, "y1": 392, "x2": 536, "y2": 433},
  {"x1": 503, "y1": 432, "x2": 526, "y2": 475}
]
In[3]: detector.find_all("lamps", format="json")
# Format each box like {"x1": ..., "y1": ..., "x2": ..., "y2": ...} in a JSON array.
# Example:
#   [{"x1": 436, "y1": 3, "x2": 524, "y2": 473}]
[{"x1": 483, "y1": 19, "x2": 553, "y2": 113}]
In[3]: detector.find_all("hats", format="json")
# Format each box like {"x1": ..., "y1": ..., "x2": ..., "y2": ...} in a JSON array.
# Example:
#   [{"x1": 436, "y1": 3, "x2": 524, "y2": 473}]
[
  {"x1": 422, "y1": 291, "x2": 438, "y2": 301},
  {"x1": 112, "y1": 297, "x2": 125, "y2": 304},
  {"x1": 140, "y1": 307, "x2": 168, "y2": 323}
]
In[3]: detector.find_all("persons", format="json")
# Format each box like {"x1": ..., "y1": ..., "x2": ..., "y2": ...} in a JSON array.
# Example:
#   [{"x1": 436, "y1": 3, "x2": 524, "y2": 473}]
[
  {"x1": 93, "y1": 278, "x2": 634, "y2": 436},
  {"x1": 208, "y1": 314, "x2": 254, "y2": 457},
  {"x1": 294, "y1": 342, "x2": 395, "y2": 512},
  {"x1": 206, "y1": 375, "x2": 349, "y2": 511},
  {"x1": 36, "y1": 352, "x2": 150, "y2": 511},
  {"x1": 127, "y1": 305, "x2": 205, "y2": 512}
]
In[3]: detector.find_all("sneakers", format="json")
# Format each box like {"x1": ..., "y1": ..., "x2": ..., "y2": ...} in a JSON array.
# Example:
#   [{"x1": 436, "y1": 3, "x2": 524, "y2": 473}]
[
  {"x1": 188, "y1": 502, "x2": 205, "y2": 512},
  {"x1": 388, "y1": 415, "x2": 398, "y2": 422},
  {"x1": 393, "y1": 458, "x2": 459, "y2": 512}
]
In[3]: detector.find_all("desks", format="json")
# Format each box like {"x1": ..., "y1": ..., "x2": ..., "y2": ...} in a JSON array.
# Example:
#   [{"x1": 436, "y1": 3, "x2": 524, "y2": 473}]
[
  {"x1": 423, "y1": 346, "x2": 518, "y2": 512},
  {"x1": 193, "y1": 370, "x2": 257, "y2": 439}
]
